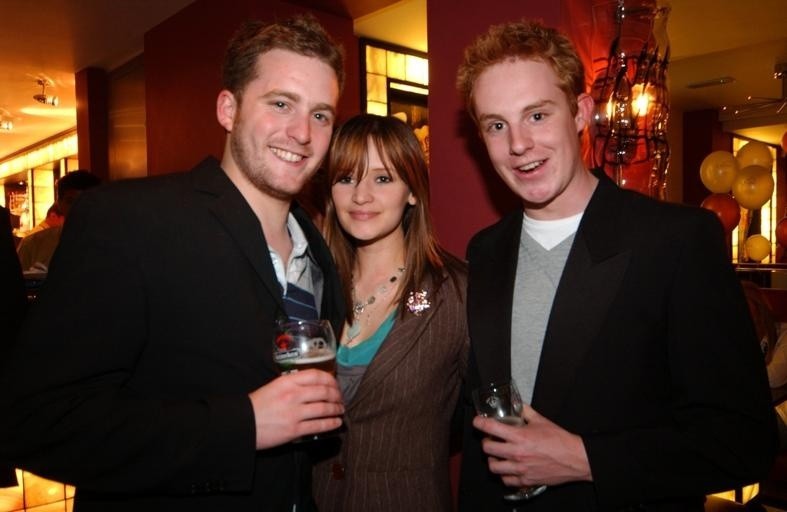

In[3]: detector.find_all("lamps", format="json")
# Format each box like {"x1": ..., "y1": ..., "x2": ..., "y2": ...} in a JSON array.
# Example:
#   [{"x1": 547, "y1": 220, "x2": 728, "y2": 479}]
[{"x1": 601, "y1": 3, "x2": 672, "y2": 199}]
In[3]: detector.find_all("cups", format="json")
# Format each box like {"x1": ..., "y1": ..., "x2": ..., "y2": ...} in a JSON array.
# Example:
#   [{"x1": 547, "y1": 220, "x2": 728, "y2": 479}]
[{"x1": 271, "y1": 318, "x2": 337, "y2": 445}]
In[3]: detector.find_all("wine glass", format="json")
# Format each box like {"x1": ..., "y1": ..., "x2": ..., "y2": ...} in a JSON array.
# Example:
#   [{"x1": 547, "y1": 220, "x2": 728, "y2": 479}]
[{"x1": 471, "y1": 375, "x2": 547, "y2": 502}]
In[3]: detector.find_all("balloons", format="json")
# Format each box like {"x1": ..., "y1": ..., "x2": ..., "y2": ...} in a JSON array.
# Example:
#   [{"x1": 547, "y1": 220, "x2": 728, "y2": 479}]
[
  {"x1": 732, "y1": 165, "x2": 774, "y2": 210},
  {"x1": 735, "y1": 141, "x2": 773, "y2": 173},
  {"x1": 700, "y1": 193, "x2": 740, "y2": 236},
  {"x1": 746, "y1": 234, "x2": 769, "y2": 261},
  {"x1": 700, "y1": 151, "x2": 740, "y2": 194}
]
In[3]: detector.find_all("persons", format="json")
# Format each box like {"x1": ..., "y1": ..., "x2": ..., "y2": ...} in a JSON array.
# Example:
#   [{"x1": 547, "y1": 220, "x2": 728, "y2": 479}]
[
  {"x1": 15, "y1": 171, "x2": 98, "y2": 271},
  {"x1": 1, "y1": 205, "x2": 30, "y2": 487},
  {"x1": 16, "y1": 201, "x2": 64, "y2": 253},
  {"x1": 310, "y1": 113, "x2": 470, "y2": 511},
  {"x1": 32, "y1": 15, "x2": 345, "y2": 509},
  {"x1": 739, "y1": 280, "x2": 787, "y2": 407},
  {"x1": 456, "y1": 16, "x2": 782, "y2": 510}
]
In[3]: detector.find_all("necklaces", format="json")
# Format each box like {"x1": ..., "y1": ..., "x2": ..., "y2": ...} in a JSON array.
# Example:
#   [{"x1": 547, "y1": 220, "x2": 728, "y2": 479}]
[
  {"x1": 347, "y1": 256, "x2": 405, "y2": 347},
  {"x1": 39, "y1": 224, "x2": 46, "y2": 230}
]
